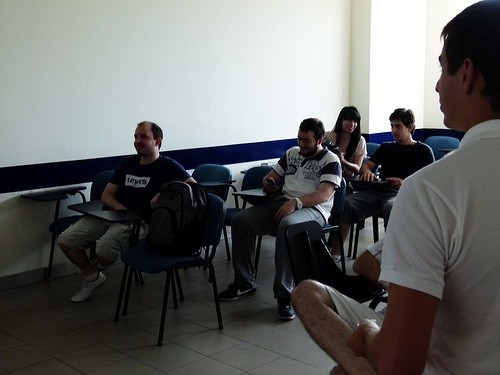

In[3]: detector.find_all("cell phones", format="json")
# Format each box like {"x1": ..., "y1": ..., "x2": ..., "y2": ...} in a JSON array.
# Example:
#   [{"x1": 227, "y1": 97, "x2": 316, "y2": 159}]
[{"x1": 265, "y1": 177, "x2": 277, "y2": 188}]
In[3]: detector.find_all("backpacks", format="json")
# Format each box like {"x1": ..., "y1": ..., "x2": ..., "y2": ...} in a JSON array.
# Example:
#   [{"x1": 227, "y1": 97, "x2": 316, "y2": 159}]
[{"x1": 148, "y1": 180, "x2": 207, "y2": 241}]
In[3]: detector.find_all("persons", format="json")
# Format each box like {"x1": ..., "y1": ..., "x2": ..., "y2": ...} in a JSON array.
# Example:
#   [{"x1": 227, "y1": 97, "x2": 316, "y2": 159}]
[
  {"x1": 58, "y1": 121, "x2": 199, "y2": 303},
  {"x1": 290, "y1": 0, "x2": 500, "y2": 375},
  {"x1": 323, "y1": 105, "x2": 434, "y2": 287},
  {"x1": 217, "y1": 118, "x2": 342, "y2": 321}
]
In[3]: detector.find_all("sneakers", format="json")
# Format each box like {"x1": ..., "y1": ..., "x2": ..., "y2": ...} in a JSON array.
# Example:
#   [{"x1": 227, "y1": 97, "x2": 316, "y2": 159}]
[
  {"x1": 77, "y1": 261, "x2": 106, "y2": 274},
  {"x1": 219, "y1": 284, "x2": 256, "y2": 301},
  {"x1": 278, "y1": 300, "x2": 295, "y2": 319},
  {"x1": 71, "y1": 272, "x2": 106, "y2": 303}
]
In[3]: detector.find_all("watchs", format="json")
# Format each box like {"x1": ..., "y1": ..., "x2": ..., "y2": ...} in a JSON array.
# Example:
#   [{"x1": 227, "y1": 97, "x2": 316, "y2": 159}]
[{"x1": 293, "y1": 197, "x2": 303, "y2": 210}]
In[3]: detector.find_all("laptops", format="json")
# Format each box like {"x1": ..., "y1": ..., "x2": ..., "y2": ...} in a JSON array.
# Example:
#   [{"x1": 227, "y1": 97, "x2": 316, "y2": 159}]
[{"x1": 348, "y1": 178, "x2": 398, "y2": 195}]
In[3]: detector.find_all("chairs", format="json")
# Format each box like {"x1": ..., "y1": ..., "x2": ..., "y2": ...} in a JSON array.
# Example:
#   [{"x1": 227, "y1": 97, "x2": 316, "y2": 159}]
[{"x1": 18, "y1": 135, "x2": 460, "y2": 346}]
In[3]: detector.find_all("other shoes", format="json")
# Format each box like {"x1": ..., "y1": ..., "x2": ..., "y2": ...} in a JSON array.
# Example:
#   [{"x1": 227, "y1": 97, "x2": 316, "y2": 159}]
[{"x1": 333, "y1": 255, "x2": 343, "y2": 270}]
